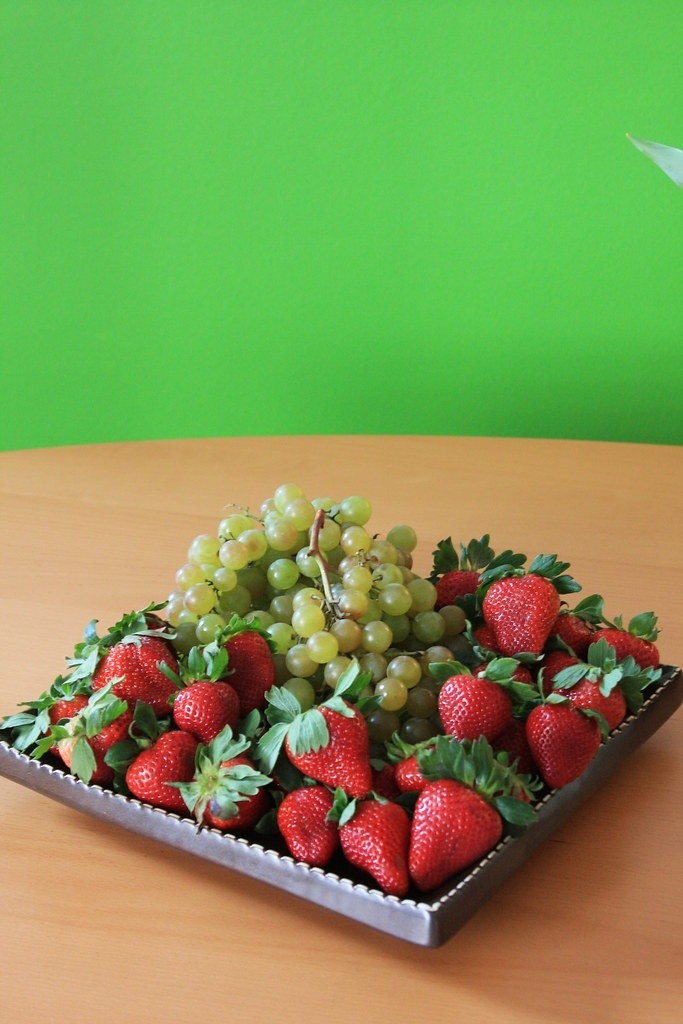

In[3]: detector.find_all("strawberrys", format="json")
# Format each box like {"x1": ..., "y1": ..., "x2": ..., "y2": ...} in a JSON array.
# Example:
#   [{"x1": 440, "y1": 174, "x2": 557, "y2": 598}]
[{"x1": 1, "y1": 531, "x2": 665, "y2": 898}]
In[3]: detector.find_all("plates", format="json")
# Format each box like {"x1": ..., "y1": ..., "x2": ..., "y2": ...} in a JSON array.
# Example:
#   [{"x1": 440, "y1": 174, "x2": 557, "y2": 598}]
[{"x1": 1, "y1": 662, "x2": 683, "y2": 949}]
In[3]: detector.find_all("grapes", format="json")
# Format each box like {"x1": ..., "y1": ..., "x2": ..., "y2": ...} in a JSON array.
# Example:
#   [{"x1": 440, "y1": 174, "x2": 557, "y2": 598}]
[{"x1": 164, "y1": 483, "x2": 471, "y2": 742}]
